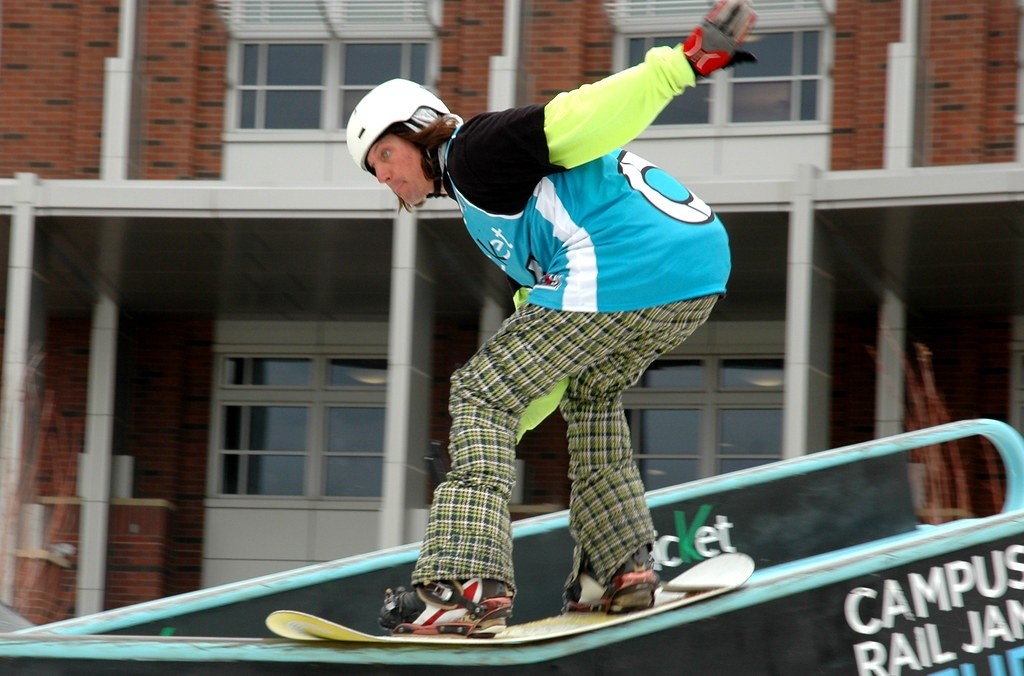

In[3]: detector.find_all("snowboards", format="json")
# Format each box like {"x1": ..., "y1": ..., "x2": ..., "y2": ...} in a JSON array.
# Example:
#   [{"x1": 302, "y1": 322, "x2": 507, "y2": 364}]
[{"x1": 265, "y1": 550, "x2": 756, "y2": 648}]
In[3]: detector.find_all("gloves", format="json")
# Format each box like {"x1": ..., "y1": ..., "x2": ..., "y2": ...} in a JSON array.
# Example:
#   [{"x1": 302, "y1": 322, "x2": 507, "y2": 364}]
[{"x1": 685, "y1": 0, "x2": 760, "y2": 78}]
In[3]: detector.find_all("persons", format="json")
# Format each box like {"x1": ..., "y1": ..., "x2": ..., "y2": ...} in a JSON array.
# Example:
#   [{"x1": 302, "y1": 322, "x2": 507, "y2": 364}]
[{"x1": 345, "y1": 2, "x2": 763, "y2": 639}]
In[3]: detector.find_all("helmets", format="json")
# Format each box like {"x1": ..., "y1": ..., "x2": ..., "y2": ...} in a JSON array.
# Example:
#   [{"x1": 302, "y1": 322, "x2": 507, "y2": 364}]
[{"x1": 346, "y1": 78, "x2": 451, "y2": 171}]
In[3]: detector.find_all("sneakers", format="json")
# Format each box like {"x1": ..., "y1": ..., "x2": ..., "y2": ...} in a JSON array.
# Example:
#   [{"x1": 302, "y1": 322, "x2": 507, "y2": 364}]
[
  {"x1": 378, "y1": 578, "x2": 514, "y2": 638},
  {"x1": 560, "y1": 568, "x2": 659, "y2": 613}
]
